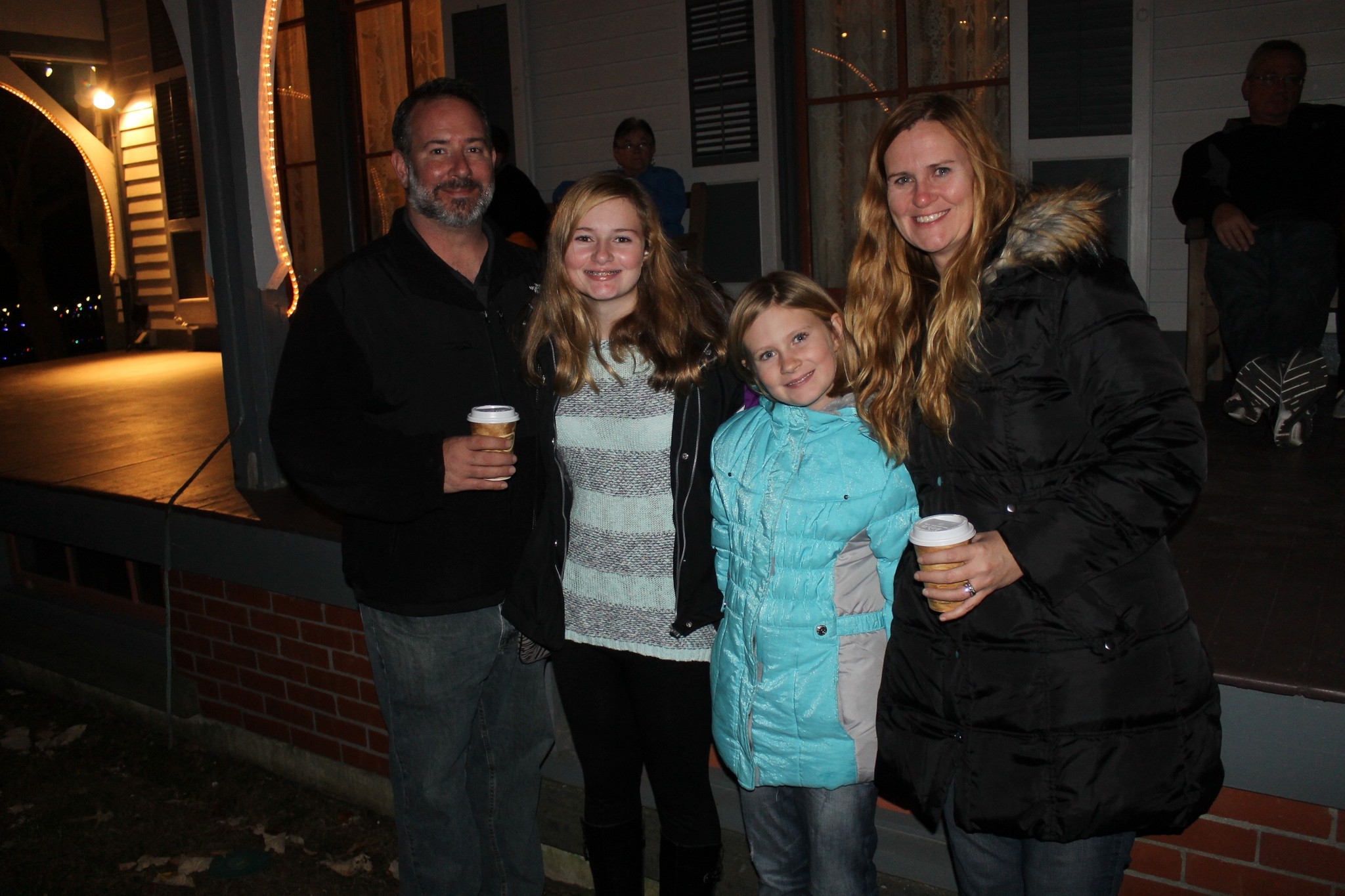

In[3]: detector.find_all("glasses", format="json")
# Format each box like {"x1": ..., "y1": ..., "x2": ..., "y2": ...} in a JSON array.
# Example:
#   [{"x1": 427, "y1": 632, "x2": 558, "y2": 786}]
[
  {"x1": 617, "y1": 141, "x2": 651, "y2": 151},
  {"x1": 1250, "y1": 73, "x2": 1305, "y2": 87}
]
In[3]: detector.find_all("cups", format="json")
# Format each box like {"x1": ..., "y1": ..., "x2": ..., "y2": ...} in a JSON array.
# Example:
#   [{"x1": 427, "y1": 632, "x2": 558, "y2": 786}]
[
  {"x1": 910, "y1": 513, "x2": 978, "y2": 612},
  {"x1": 466, "y1": 405, "x2": 520, "y2": 481}
]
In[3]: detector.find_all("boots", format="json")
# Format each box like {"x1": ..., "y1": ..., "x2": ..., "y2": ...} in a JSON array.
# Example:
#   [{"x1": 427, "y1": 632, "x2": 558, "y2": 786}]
[
  {"x1": 657, "y1": 827, "x2": 725, "y2": 896},
  {"x1": 580, "y1": 811, "x2": 646, "y2": 896}
]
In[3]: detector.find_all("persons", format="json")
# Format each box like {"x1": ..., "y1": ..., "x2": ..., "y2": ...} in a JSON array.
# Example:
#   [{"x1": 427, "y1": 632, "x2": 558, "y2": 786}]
[
  {"x1": 499, "y1": 170, "x2": 760, "y2": 896},
  {"x1": 1172, "y1": 40, "x2": 1345, "y2": 449},
  {"x1": 265, "y1": 75, "x2": 567, "y2": 896},
  {"x1": 386, "y1": 115, "x2": 688, "y2": 258},
  {"x1": 837, "y1": 93, "x2": 1227, "y2": 896},
  {"x1": 704, "y1": 269, "x2": 920, "y2": 895}
]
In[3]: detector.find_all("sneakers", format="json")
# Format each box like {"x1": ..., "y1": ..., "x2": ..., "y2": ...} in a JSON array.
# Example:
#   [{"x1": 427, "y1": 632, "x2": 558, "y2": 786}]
[
  {"x1": 1332, "y1": 390, "x2": 1345, "y2": 420},
  {"x1": 1222, "y1": 352, "x2": 1284, "y2": 427},
  {"x1": 1268, "y1": 343, "x2": 1328, "y2": 455}
]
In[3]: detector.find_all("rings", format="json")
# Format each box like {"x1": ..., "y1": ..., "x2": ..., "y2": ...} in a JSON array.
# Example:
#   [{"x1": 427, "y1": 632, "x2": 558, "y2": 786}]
[{"x1": 963, "y1": 582, "x2": 977, "y2": 598}]
